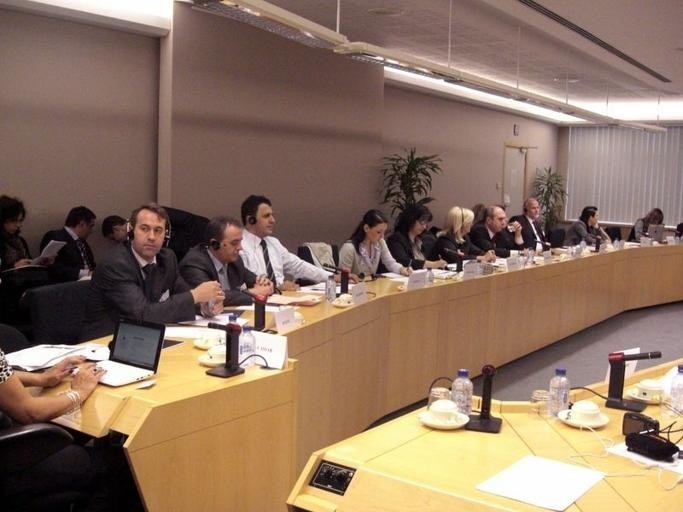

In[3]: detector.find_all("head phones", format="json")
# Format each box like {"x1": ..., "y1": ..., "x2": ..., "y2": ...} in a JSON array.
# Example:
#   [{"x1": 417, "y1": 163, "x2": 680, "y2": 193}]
[
  {"x1": 127, "y1": 209, "x2": 172, "y2": 242},
  {"x1": 247, "y1": 196, "x2": 259, "y2": 224},
  {"x1": 206, "y1": 241, "x2": 223, "y2": 249}
]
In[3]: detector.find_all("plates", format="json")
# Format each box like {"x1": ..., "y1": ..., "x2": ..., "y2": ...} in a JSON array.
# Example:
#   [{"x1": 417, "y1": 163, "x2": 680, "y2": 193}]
[
  {"x1": 332, "y1": 301, "x2": 353, "y2": 308},
  {"x1": 558, "y1": 410, "x2": 609, "y2": 428},
  {"x1": 418, "y1": 413, "x2": 470, "y2": 429},
  {"x1": 191, "y1": 337, "x2": 225, "y2": 367},
  {"x1": 627, "y1": 389, "x2": 661, "y2": 404}
]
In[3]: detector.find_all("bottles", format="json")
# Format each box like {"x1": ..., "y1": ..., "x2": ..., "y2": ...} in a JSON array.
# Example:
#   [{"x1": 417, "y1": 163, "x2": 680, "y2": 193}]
[
  {"x1": 228, "y1": 315, "x2": 236, "y2": 324},
  {"x1": 670, "y1": 364, "x2": 682, "y2": 421},
  {"x1": 452, "y1": 367, "x2": 472, "y2": 416},
  {"x1": 579, "y1": 235, "x2": 587, "y2": 257},
  {"x1": 425, "y1": 266, "x2": 433, "y2": 288},
  {"x1": 238, "y1": 325, "x2": 255, "y2": 366},
  {"x1": 548, "y1": 367, "x2": 569, "y2": 419},
  {"x1": 528, "y1": 247, "x2": 534, "y2": 263},
  {"x1": 324, "y1": 276, "x2": 336, "y2": 304}
]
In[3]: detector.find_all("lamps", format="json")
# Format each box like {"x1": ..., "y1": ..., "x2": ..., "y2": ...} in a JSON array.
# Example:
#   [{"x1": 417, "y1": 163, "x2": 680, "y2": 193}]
[
  {"x1": 183, "y1": 0, "x2": 349, "y2": 50},
  {"x1": 336, "y1": 0, "x2": 665, "y2": 134}
]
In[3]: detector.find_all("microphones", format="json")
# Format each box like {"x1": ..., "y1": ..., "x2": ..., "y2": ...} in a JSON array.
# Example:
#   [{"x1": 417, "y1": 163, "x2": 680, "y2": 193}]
[
  {"x1": 323, "y1": 263, "x2": 350, "y2": 295},
  {"x1": 606, "y1": 346, "x2": 661, "y2": 411},
  {"x1": 442, "y1": 247, "x2": 463, "y2": 272},
  {"x1": 534, "y1": 239, "x2": 552, "y2": 252},
  {"x1": 205, "y1": 321, "x2": 244, "y2": 379},
  {"x1": 242, "y1": 286, "x2": 266, "y2": 333},
  {"x1": 466, "y1": 363, "x2": 502, "y2": 434},
  {"x1": 590, "y1": 233, "x2": 601, "y2": 252}
]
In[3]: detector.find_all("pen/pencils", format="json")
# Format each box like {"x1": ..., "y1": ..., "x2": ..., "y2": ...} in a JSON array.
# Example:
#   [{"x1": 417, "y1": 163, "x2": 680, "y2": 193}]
[
  {"x1": 438, "y1": 254, "x2": 445, "y2": 270},
  {"x1": 407, "y1": 259, "x2": 412, "y2": 271}
]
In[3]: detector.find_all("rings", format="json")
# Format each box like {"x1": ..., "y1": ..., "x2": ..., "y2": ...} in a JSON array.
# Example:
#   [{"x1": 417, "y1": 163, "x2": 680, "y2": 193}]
[{"x1": 91, "y1": 368, "x2": 99, "y2": 374}]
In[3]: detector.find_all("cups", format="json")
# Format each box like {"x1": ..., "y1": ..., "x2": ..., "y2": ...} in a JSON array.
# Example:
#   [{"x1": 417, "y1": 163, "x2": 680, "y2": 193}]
[
  {"x1": 510, "y1": 250, "x2": 518, "y2": 263},
  {"x1": 569, "y1": 401, "x2": 601, "y2": 425},
  {"x1": 426, "y1": 386, "x2": 451, "y2": 410},
  {"x1": 632, "y1": 379, "x2": 661, "y2": 399},
  {"x1": 497, "y1": 263, "x2": 505, "y2": 272},
  {"x1": 334, "y1": 293, "x2": 352, "y2": 304},
  {"x1": 506, "y1": 223, "x2": 516, "y2": 231},
  {"x1": 530, "y1": 389, "x2": 551, "y2": 418},
  {"x1": 428, "y1": 400, "x2": 458, "y2": 422}
]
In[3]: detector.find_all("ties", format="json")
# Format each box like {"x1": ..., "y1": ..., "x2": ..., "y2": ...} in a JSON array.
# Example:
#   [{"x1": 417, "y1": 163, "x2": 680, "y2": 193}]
[{"x1": 259, "y1": 239, "x2": 277, "y2": 288}]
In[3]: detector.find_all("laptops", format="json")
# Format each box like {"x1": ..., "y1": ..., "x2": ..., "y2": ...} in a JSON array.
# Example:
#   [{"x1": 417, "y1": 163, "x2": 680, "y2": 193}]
[
  {"x1": 648, "y1": 224, "x2": 664, "y2": 241},
  {"x1": 72, "y1": 316, "x2": 165, "y2": 389}
]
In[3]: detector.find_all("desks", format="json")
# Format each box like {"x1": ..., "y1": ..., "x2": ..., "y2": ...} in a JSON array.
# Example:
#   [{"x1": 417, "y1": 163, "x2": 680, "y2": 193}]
[
  {"x1": 488, "y1": 220, "x2": 683, "y2": 369},
  {"x1": 0, "y1": 263, "x2": 488, "y2": 512},
  {"x1": 282, "y1": 361, "x2": 682, "y2": 512}
]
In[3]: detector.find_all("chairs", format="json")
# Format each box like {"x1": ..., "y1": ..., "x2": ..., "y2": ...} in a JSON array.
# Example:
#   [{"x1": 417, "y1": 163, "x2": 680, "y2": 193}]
[{"x1": 1, "y1": 222, "x2": 449, "y2": 293}]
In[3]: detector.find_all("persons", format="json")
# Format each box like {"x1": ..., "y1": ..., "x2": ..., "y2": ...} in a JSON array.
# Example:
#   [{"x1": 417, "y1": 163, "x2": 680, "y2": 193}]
[
  {"x1": 0, "y1": 196, "x2": 361, "y2": 344},
  {"x1": 626, "y1": 208, "x2": 663, "y2": 242},
  {"x1": 339, "y1": 195, "x2": 551, "y2": 278},
  {"x1": 0, "y1": 348, "x2": 107, "y2": 503},
  {"x1": 561, "y1": 206, "x2": 612, "y2": 247}
]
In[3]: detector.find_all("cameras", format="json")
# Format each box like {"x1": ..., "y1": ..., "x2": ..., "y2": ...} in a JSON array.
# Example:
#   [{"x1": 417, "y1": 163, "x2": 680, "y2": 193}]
[{"x1": 622, "y1": 411, "x2": 659, "y2": 436}]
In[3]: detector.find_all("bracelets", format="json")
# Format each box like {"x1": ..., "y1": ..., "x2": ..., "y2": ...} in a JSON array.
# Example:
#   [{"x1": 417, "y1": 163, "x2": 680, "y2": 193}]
[{"x1": 57, "y1": 390, "x2": 82, "y2": 415}]
